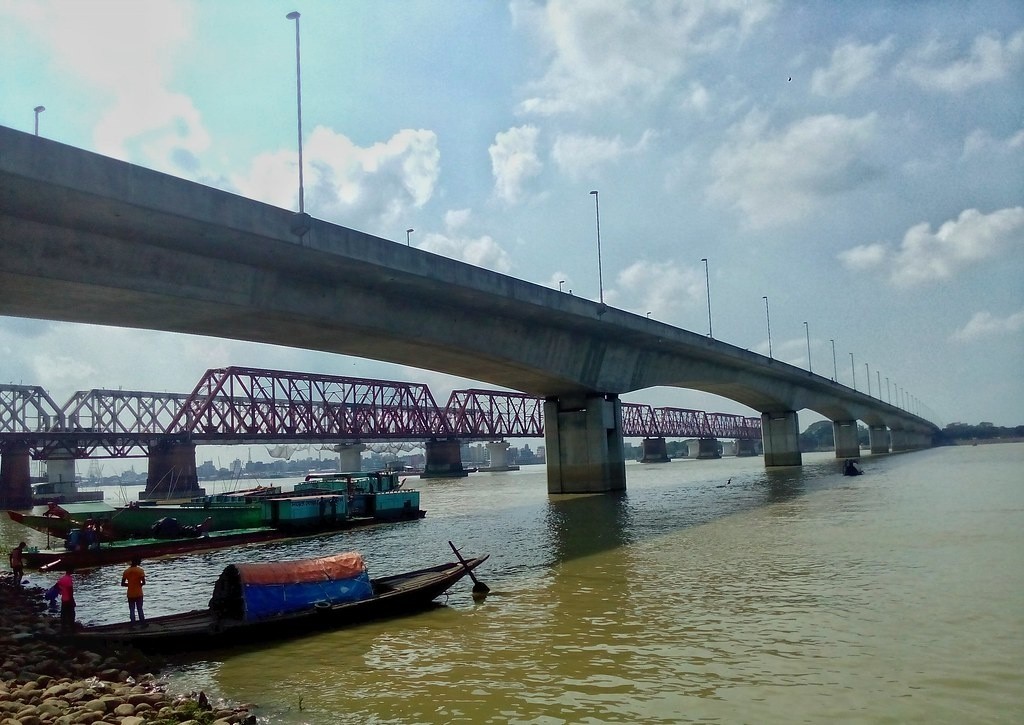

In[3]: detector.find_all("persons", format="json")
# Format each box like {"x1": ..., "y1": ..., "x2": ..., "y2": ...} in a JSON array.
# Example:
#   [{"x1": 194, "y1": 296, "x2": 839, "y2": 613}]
[
  {"x1": 10, "y1": 542, "x2": 26, "y2": 586},
  {"x1": 348, "y1": 496, "x2": 352, "y2": 515},
  {"x1": 57, "y1": 566, "x2": 76, "y2": 633},
  {"x1": 330, "y1": 496, "x2": 337, "y2": 520},
  {"x1": 121, "y1": 556, "x2": 149, "y2": 628},
  {"x1": 319, "y1": 497, "x2": 326, "y2": 518}
]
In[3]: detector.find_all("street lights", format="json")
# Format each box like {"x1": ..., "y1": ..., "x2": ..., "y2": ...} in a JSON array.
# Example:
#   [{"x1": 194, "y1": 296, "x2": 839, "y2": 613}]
[
  {"x1": 287, "y1": 12, "x2": 303, "y2": 212},
  {"x1": 877, "y1": 371, "x2": 881, "y2": 400},
  {"x1": 647, "y1": 312, "x2": 651, "y2": 318},
  {"x1": 906, "y1": 392, "x2": 921, "y2": 417},
  {"x1": 407, "y1": 229, "x2": 414, "y2": 247},
  {"x1": 763, "y1": 297, "x2": 772, "y2": 358},
  {"x1": 900, "y1": 388, "x2": 904, "y2": 410},
  {"x1": 886, "y1": 378, "x2": 890, "y2": 404},
  {"x1": 35, "y1": 106, "x2": 45, "y2": 136},
  {"x1": 590, "y1": 191, "x2": 603, "y2": 304},
  {"x1": 830, "y1": 340, "x2": 837, "y2": 382},
  {"x1": 865, "y1": 363, "x2": 870, "y2": 395},
  {"x1": 701, "y1": 259, "x2": 712, "y2": 338},
  {"x1": 894, "y1": 384, "x2": 898, "y2": 407},
  {"x1": 804, "y1": 322, "x2": 811, "y2": 372},
  {"x1": 849, "y1": 353, "x2": 855, "y2": 389},
  {"x1": 560, "y1": 281, "x2": 564, "y2": 292}
]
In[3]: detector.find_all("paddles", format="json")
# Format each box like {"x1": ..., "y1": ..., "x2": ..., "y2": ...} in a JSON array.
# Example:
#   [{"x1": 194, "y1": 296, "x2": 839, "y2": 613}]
[{"x1": 448, "y1": 540, "x2": 491, "y2": 594}]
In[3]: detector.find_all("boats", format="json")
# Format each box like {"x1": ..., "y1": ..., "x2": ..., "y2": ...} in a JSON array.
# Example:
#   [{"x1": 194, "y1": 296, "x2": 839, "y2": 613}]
[
  {"x1": 6, "y1": 470, "x2": 427, "y2": 566},
  {"x1": 845, "y1": 458, "x2": 860, "y2": 476},
  {"x1": 65, "y1": 553, "x2": 490, "y2": 656}
]
